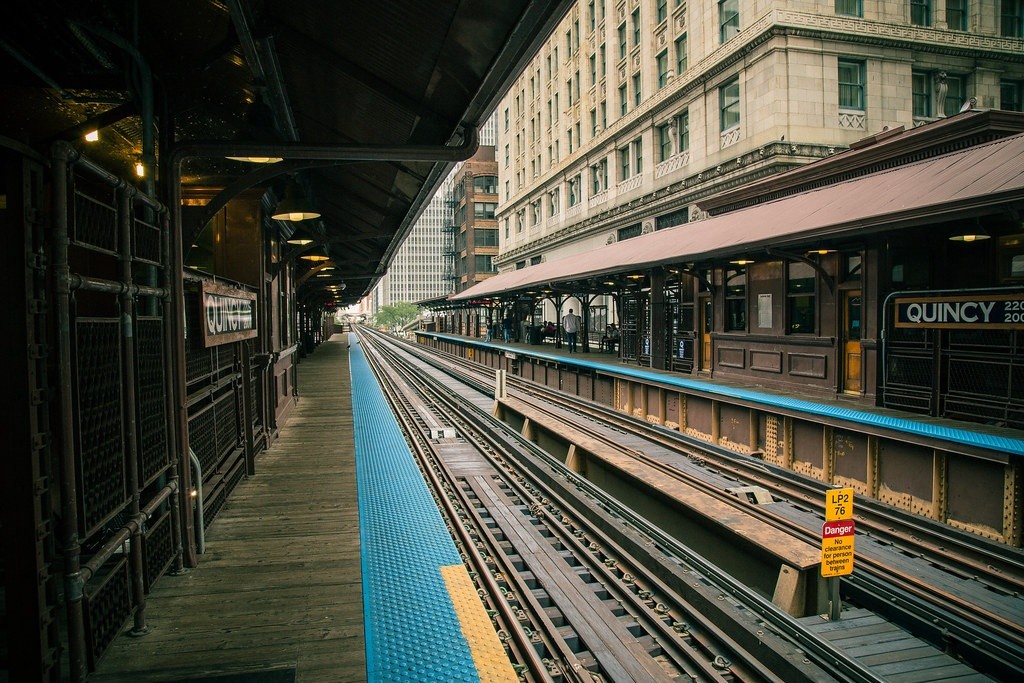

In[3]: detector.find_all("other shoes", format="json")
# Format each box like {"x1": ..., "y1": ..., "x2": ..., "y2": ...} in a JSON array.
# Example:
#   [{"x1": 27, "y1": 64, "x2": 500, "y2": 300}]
[
  {"x1": 569, "y1": 351, "x2": 577, "y2": 353},
  {"x1": 607, "y1": 351, "x2": 612, "y2": 354}
]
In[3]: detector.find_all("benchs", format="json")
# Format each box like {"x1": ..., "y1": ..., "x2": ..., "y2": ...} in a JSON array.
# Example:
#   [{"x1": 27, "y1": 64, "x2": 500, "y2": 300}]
[{"x1": 539, "y1": 328, "x2": 561, "y2": 345}]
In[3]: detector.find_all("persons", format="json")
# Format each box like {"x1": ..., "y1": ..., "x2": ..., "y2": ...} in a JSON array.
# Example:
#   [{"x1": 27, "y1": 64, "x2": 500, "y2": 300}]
[
  {"x1": 502, "y1": 314, "x2": 512, "y2": 343},
  {"x1": 541, "y1": 321, "x2": 555, "y2": 341},
  {"x1": 484, "y1": 319, "x2": 492, "y2": 342},
  {"x1": 563, "y1": 308, "x2": 580, "y2": 353},
  {"x1": 598, "y1": 323, "x2": 620, "y2": 353}
]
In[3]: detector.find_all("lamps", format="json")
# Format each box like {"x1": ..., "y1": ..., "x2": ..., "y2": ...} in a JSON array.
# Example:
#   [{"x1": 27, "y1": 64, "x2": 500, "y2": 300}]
[
  {"x1": 222, "y1": 93, "x2": 283, "y2": 165},
  {"x1": 300, "y1": 244, "x2": 329, "y2": 261},
  {"x1": 269, "y1": 175, "x2": 323, "y2": 221},
  {"x1": 285, "y1": 225, "x2": 315, "y2": 244}
]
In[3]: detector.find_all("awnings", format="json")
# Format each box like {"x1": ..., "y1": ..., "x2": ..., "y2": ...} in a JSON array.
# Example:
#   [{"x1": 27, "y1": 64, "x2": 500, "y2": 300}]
[
  {"x1": 228, "y1": 0, "x2": 578, "y2": 307},
  {"x1": 446, "y1": 132, "x2": 1024, "y2": 302}
]
